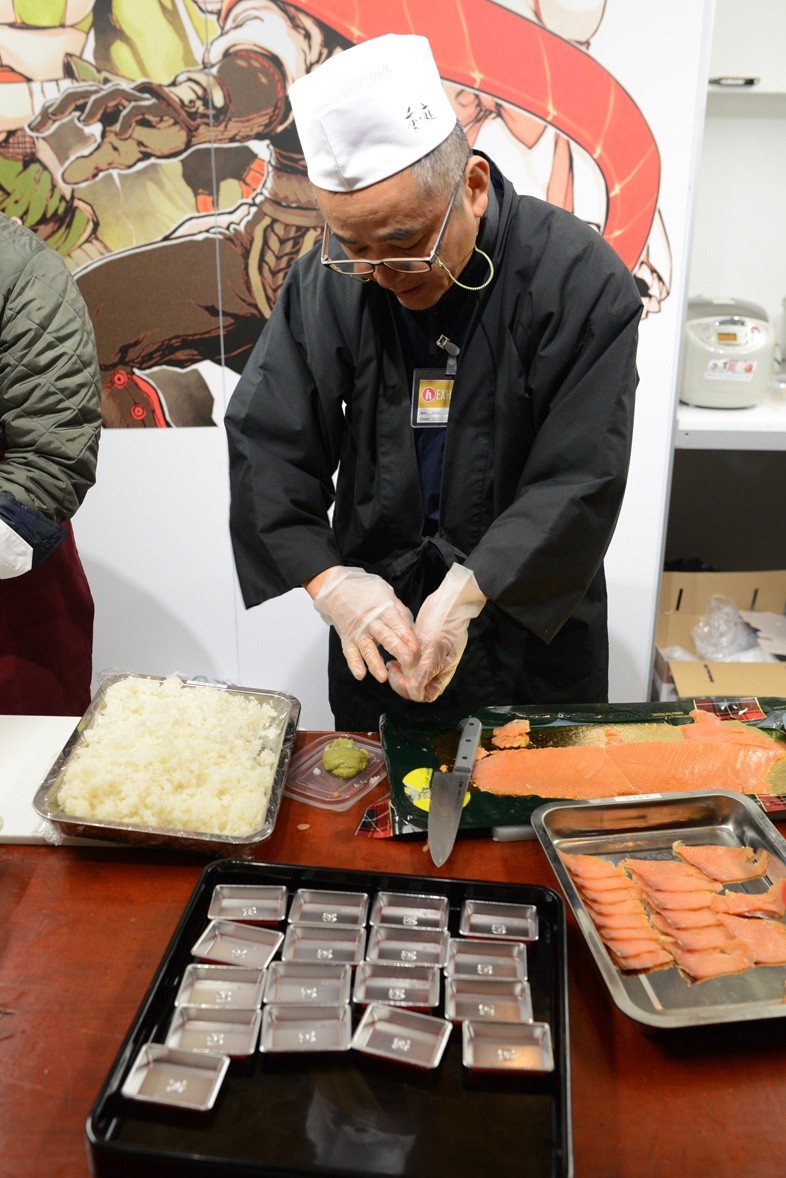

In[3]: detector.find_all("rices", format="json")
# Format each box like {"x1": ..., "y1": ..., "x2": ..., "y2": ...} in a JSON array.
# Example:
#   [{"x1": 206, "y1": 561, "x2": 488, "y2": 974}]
[{"x1": 56, "y1": 676, "x2": 279, "y2": 837}]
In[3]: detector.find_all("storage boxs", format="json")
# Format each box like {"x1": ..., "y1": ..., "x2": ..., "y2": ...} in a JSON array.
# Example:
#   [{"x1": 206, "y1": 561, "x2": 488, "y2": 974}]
[{"x1": 651, "y1": 570, "x2": 786, "y2": 742}]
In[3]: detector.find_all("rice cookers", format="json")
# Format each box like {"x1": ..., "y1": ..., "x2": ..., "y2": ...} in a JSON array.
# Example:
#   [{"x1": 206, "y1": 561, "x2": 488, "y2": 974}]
[{"x1": 677, "y1": 294, "x2": 775, "y2": 410}]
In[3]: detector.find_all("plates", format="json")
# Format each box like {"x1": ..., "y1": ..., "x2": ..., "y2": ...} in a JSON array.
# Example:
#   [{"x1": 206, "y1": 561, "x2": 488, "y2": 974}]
[
  {"x1": 287, "y1": 888, "x2": 369, "y2": 928},
  {"x1": 352, "y1": 962, "x2": 440, "y2": 1012},
  {"x1": 444, "y1": 938, "x2": 528, "y2": 980},
  {"x1": 365, "y1": 926, "x2": 451, "y2": 968},
  {"x1": 352, "y1": 1001, "x2": 453, "y2": 1072},
  {"x1": 462, "y1": 1020, "x2": 555, "y2": 1077},
  {"x1": 164, "y1": 1004, "x2": 263, "y2": 1062},
  {"x1": 207, "y1": 884, "x2": 287, "y2": 927},
  {"x1": 257, "y1": 1004, "x2": 351, "y2": 1052},
  {"x1": 280, "y1": 923, "x2": 367, "y2": 973},
  {"x1": 173, "y1": 965, "x2": 268, "y2": 1008},
  {"x1": 263, "y1": 961, "x2": 352, "y2": 1004},
  {"x1": 445, "y1": 976, "x2": 534, "y2": 1026},
  {"x1": 190, "y1": 918, "x2": 285, "y2": 969},
  {"x1": 121, "y1": 1043, "x2": 230, "y2": 1110},
  {"x1": 458, "y1": 900, "x2": 538, "y2": 946},
  {"x1": 370, "y1": 891, "x2": 449, "y2": 929}
]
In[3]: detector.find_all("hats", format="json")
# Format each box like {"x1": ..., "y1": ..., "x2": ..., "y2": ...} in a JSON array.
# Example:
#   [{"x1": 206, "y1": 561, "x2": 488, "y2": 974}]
[{"x1": 285, "y1": 32, "x2": 456, "y2": 193}]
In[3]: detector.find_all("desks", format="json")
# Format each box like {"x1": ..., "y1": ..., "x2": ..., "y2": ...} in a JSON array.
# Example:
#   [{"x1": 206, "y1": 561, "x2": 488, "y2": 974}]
[{"x1": 0, "y1": 732, "x2": 786, "y2": 1178}]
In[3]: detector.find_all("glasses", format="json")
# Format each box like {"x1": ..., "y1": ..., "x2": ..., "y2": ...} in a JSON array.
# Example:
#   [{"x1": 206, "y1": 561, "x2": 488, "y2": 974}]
[{"x1": 321, "y1": 196, "x2": 456, "y2": 277}]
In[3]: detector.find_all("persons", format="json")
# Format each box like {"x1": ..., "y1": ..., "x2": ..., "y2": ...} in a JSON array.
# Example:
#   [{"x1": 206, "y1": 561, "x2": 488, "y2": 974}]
[
  {"x1": 221, "y1": 33, "x2": 644, "y2": 730},
  {"x1": 0, "y1": 210, "x2": 103, "y2": 716}
]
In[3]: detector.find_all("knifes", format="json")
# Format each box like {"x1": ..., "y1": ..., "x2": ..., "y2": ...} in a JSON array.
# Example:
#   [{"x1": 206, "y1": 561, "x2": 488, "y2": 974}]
[{"x1": 427, "y1": 717, "x2": 481, "y2": 870}]
